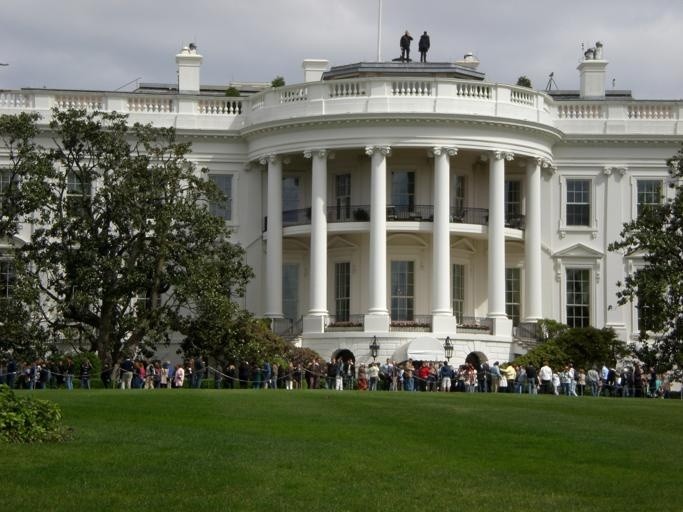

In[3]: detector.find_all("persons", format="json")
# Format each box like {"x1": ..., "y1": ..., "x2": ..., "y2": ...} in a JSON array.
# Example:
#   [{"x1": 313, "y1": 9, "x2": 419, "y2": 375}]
[
  {"x1": 400, "y1": 30, "x2": 413, "y2": 62},
  {"x1": 1, "y1": 356, "x2": 327, "y2": 389},
  {"x1": 322, "y1": 357, "x2": 666, "y2": 401},
  {"x1": 419, "y1": 31, "x2": 430, "y2": 62}
]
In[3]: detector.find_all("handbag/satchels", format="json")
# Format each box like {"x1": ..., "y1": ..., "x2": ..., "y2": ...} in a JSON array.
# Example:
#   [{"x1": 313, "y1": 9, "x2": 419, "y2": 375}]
[
  {"x1": 574, "y1": 370, "x2": 579, "y2": 380},
  {"x1": 154, "y1": 374, "x2": 161, "y2": 383}
]
